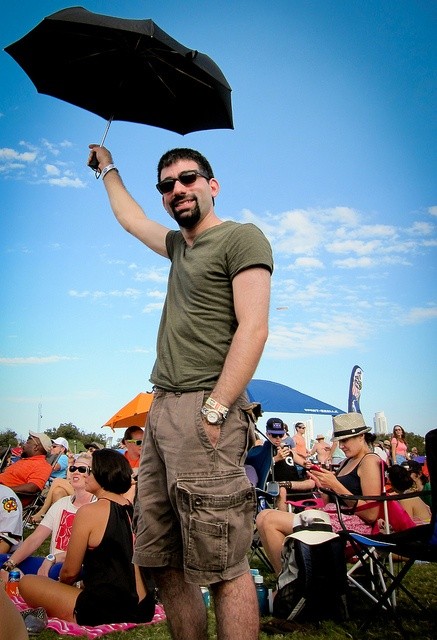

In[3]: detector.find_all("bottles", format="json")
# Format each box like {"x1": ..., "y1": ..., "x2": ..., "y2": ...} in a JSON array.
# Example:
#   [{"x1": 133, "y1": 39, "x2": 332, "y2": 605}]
[{"x1": 254, "y1": 574, "x2": 266, "y2": 611}]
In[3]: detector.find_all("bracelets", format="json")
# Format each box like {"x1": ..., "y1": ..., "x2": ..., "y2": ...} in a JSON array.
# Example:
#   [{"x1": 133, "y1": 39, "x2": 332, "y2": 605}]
[
  {"x1": 3, "y1": 559, "x2": 16, "y2": 568},
  {"x1": 205, "y1": 396, "x2": 230, "y2": 418},
  {"x1": 101, "y1": 164, "x2": 119, "y2": 180}
]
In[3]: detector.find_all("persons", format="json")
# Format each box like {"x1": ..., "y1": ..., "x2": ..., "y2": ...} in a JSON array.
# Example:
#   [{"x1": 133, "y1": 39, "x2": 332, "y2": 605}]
[
  {"x1": 292, "y1": 421, "x2": 307, "y2": 457},
  {"x1": 0, "y1": 430, "x2": 52, "y2": 508},
  {"x1": 87, "y1": 148, "x2": 275, "y2": 639},
  {"x1": 255, "y1": 412, "x2": 384, "y2": 575},
  {"x1": 123, "y1": 425, "x2": 145, "y2": 477},
  {"x1": 391, "y1": 424, "x2": 408, "y2": 466},
  {"x1": 1, "y1": 452, "x2": 98, "y2": 577},
  {"x1": 405, "y1": 446, "x2": 420, "y2": 460},
  {"x1": 1, "y1": 480, "x2": 23, "y2": 555},
  {"x1": 398, "y1": 461, "x2": 431, "y2": 492},
  {"x1": 48, "y1": 437, "x2": 69, "y2": 481},
  {"x1": 18, "y1": 448, "x2": 156, "y2": 627},
  {"x1": 0, "y1": 586, "x2": 29, "y2": 639},
  {"x1": 380, "y1": 439, "x2": 392, "y2": 455},
  {"x1": 309, "y1": 434, "x2": 332, "y2": 466},
  {"x1": 387, "y1": 465, "x2": 433, "y2": 525},
  {"x1": 266, "y1": 417, "x2": 316, "y2": 511}
]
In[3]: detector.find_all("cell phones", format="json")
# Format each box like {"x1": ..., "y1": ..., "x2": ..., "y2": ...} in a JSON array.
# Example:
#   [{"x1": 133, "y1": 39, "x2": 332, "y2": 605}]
[
  {"x1": 310, "y1": 464, "x2": 322, "y2": 472},
  {"x1": 282, "y1": 445, "x2": 290, "y2": 450}
]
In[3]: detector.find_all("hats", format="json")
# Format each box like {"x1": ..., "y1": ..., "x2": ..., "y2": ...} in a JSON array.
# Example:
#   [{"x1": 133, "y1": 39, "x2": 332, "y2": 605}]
[
  {"x1": 51, "y1": 437, "x2": 68, "y2": 453},
  {"x1": 29, "y1": 431, "x2": 52, "y2": 453},
  {"x1": 331, "y1": 412, "x2": 372, "y2": 442},
  {"x1": 83, "y1": 441, "x2": 104, "y2": 451},
  {"x1": 266, "y1": 417, "x2": 286, "y2": 435}
]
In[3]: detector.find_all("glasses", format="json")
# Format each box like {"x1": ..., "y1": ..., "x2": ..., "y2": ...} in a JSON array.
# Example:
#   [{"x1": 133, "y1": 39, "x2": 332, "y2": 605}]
[
  {"x1": 69, "y1": 466, "x2": 86, "y2": 473},
  {"x1": 127, "y1": 440, "x2": 142, "y2": 445},
  {"x1": 88, "y1": 448, "x2": 96, "y2": 452},
  {"x1": 156, "y1": 170, "x2": 208, "y2": 194},
  {"x1": 300, "y1": 427, "x2": 306, "y2": 429},
  {"x1": 271, "y1": 433, "x2": 284, "y2": 438},
  {"x1": 86, "y1": 466, "x2": 91, "y2": 476},
  {"x1": 395, "y1": 429, "x2": 401, "y2": 431},
  {"x1": 28, "y1": 436, "x2": 38, "y2": 446},
  {"x1": 51, "y1": 444, "x2": 59, "y2": 448}
]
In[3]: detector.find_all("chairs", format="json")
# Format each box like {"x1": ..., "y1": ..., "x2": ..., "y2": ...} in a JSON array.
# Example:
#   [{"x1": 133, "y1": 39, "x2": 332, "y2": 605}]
[
  {"x1": 316, "y1": 428, "x2": 437, "y2": 639},
  {"x1": 0, "y1": 444, "x2": 11, "y2": 473},
  {"x1": 242, "y1": 440, "x2": 283, "y2": 573},
  {"x1": 285, "y1": 461, "x2": 397, "y2": 612},
  {"x1": 12, "y1": 451, "x2": 62, "y2": 529}
]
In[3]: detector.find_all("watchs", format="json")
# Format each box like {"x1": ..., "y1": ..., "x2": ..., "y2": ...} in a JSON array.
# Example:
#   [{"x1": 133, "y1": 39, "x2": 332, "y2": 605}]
[
  {"x1": 200, "y1": 406, "x2": 224, "y2": 426},
  {"x1": 46, "y1": 553, "x2": 57, "y2": 565}
]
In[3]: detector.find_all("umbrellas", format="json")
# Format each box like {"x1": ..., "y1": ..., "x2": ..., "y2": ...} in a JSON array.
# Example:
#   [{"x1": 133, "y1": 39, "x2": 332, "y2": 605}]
[
  {"x1": 3, "y1": 6, "x2": 235, "y2": 169},
  {"x1": 246, "y1": 378, "x2": 348, "y2": 416},
  {"x1": 100, "y1": 391, "x2": 156, "y2": 434}
]
